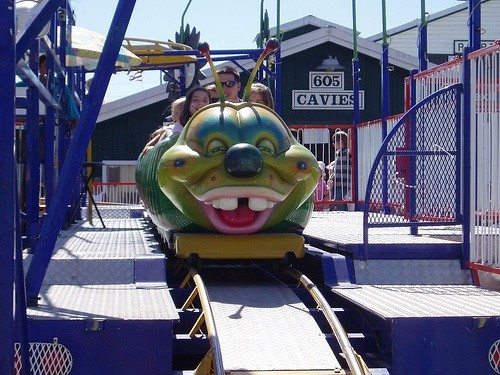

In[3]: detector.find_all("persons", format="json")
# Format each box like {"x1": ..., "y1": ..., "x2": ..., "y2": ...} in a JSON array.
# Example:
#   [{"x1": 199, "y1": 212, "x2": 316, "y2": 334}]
[
  {"x1": 330, "y1": 131, "x2": 352, "y2": 211},
  {"x1": 245, "y1": 82, "x2": 274, "y2": 109},
  {"x1": 173, "y1": 87, "x2": 212, "y2": 135},
  {"x1": 314, "y1": 161, "x2": 333, "y2": 211},
  {"x1": 90, "y1": 178, "x2": 106, "y2": 202},
  {"x1": 146, "y1": 98, "x2": 186, "y2": 147},
  {"x1": 215, "y1": 66, "x2": 242, "y2": 103}
]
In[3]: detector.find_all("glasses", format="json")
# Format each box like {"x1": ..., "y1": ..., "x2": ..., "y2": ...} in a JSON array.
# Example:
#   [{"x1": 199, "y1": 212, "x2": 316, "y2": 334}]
[{"x1": 220, "y1": 80, "x2": 239, "y2": 88}]
